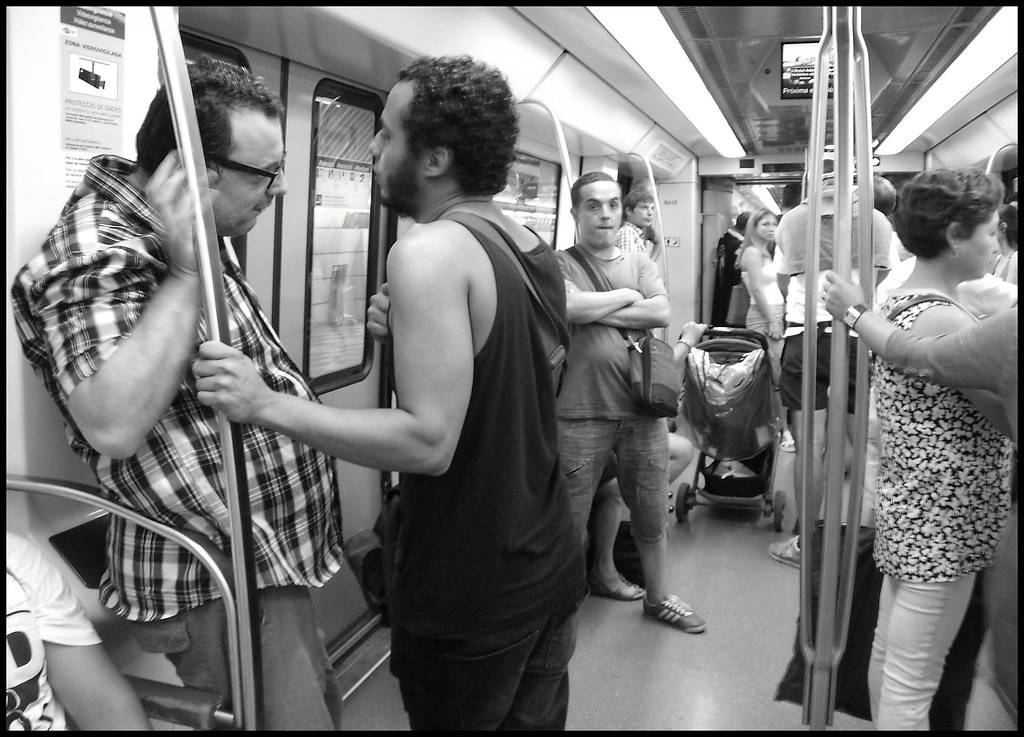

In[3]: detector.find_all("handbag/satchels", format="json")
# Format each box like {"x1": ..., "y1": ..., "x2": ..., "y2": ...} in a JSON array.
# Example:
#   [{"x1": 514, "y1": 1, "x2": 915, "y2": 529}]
[{"x1": 629, "y1": 336, "x2": 680, "y2": 418}]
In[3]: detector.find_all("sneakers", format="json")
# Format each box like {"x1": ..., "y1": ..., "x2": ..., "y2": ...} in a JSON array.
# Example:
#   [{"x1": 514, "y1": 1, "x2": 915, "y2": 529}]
[
  {"x1": 643, "y1": 595, "x2": 707, "y2": 633},
  {"x1": 768, "y1": 536, "x2": 802, "y2": 568}
]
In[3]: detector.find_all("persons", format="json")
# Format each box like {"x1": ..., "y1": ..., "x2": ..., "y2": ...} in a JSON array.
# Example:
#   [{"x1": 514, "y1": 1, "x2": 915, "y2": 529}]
[
  {"x1": 590, "y1": 322, "x2": 709, "y2": 599},
  {"x1": 710, "y1": 144, "x2": 896, "y2": 569},
  {"x1": 10, "y1": 59, "x2": 343, "y2": 731},
  {"x1": 822, "y1": 271, "x2": 1018, "y2": 439},
  {"x1": 192, "y1": 56, "x2": 590, "y2": 731},
  {"x1": 613, "y1": 187, "x2": 663, "y2": 263},
  {"x1": 554, "y1": 172, "x2": 707, "y2": 633},
  {"x1": 868, "y1": 165, "x2": 1016, "y2": 730},
  {"x1": 6, "y1": 532, "x2": 154, "y2": 731}
]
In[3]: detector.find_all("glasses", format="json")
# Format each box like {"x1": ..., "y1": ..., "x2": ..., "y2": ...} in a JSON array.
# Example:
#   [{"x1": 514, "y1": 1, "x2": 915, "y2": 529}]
[{"x1": 204, "y1": 152, "x2": 286, "y2": 189}]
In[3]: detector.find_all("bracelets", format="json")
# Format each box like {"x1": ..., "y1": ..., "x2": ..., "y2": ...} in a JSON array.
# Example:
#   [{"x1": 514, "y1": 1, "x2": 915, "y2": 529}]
[{"x1": 677, "y1": 340, "x2": 691, "y2": 354}]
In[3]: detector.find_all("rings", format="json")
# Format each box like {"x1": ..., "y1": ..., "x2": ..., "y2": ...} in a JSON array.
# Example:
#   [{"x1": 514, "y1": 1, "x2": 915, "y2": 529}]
[{"x1": 822, "y1": 292, "x2": 827, "y2": 300}]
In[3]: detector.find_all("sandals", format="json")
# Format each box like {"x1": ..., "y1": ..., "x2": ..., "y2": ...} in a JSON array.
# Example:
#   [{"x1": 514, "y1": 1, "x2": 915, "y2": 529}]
[
  {"x1": 587, "y1": 572, "x2": 645, "y2": 600},
  {"x1": 778, "y1": 431, "x2": 796, "y2": 452}
]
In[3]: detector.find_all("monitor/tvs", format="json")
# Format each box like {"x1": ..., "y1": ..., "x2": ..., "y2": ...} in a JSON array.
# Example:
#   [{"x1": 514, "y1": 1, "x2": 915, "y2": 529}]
[{"x1": 780, "y1": 39, "x2": 836, "y2": 99}]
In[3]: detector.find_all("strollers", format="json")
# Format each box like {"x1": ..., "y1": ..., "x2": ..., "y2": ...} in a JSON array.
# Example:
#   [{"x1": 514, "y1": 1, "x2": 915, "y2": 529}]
[{"x1": 673, "y1": 326, "x2": 787, "y2": 533}]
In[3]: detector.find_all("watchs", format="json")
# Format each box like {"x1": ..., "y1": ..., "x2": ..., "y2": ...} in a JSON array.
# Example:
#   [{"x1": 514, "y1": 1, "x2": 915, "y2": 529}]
[{"x1": 844, "y1": 304, "x2": 869, "y2": 329}]
